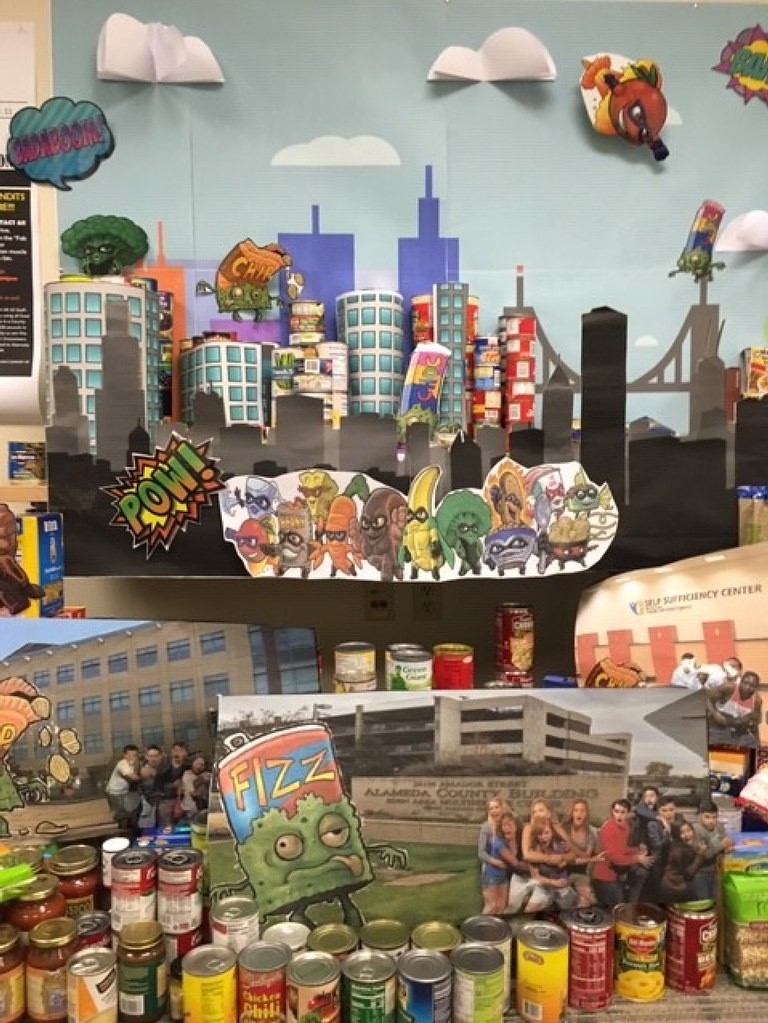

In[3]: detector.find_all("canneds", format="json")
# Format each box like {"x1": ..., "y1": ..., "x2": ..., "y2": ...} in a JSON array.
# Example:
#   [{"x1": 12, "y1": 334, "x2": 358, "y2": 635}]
[
  {"x1": 0, "y1": 810, "x2": 718, "y2": 1023},
  {"x1": 335, "y1": 603, "x2": 535, "y2": 693}
]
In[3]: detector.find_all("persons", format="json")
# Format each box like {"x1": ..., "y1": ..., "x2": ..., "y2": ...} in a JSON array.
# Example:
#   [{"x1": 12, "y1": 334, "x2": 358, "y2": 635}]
[
  {"x1": 476, "y1": 785, "x2": 735, "y2": 917},
  {"x1": 674, "y1": 651, "x2": 763, "y2": 752},
  {"x1": 105, "y1": 739, "x2": 211, "y2": 834}
]
